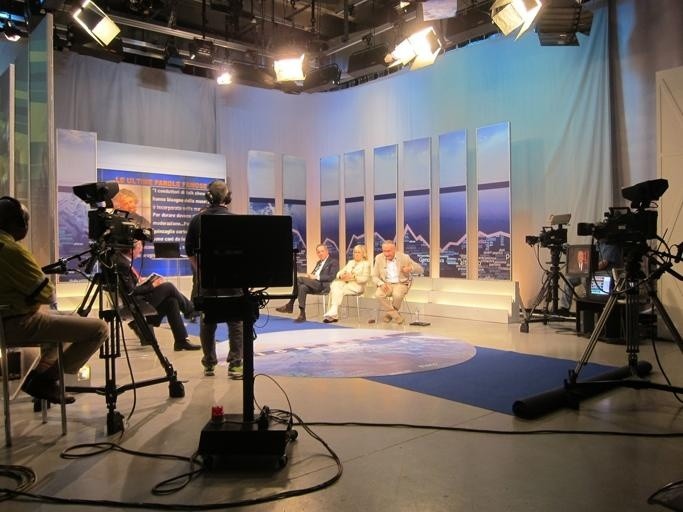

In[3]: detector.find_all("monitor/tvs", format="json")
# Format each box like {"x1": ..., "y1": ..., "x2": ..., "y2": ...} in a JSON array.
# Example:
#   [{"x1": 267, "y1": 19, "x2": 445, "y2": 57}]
[
  {"x1": 565, "y1": 245, "x2": 599, "y2": 275},
  {"x1": 586, "y1": 271, "x2": 612, "y2": 301},
  {"x1": 154, "y1": 243, "x2": 182, "y2": 258},
  {"x1": 199, "y1": 214, "x2": 293, "y2": 289}
]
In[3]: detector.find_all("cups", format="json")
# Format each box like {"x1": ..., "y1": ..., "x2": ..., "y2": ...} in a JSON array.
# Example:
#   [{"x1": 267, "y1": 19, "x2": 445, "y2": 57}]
[{"x1": 211, "y1": 404, "x2": 223, "y2": 425}]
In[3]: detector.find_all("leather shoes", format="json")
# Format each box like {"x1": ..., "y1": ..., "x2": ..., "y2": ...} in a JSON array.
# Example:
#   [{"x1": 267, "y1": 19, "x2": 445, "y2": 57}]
[
  {"x1": 24, "y1": 374, "x2": 76, "y2": 405},
  {"x1": 175, "y1": 340, "x2": 201, "y2": 351},
  {"x1": 383, "y1": 314, "x2": 391, "y2": 322},
  {"x1": 323, "y1": 317, "x2": 337, "y2": 323},
  {"x1": 276, "y1": 304, "x2": 293, "y2": 313},
  {"x1": 185, "y1": 308, "x2": 201, "y2": 319},
  {"x1": 294, "y1": 314, "x2": 306, "y2": 323},
  {"x1": 395, "y1": 316, "x2": 403, "y2": 324}
]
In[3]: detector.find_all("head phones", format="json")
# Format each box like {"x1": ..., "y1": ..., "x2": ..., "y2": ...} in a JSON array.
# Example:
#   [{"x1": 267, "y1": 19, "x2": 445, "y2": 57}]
[
  {"x1": 205, "y1": 180, "x2": 232, "y2": 205},
  {"x1": 0, "y1": 196, "x2": 25, "y2": 233}
]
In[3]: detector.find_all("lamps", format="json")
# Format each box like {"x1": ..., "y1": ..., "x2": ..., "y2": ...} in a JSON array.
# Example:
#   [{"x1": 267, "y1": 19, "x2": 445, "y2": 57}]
[
  {"x1": 389, "y1": 0, "x2": 593, "y2": 72},
  {"x1": 71, "y1": 1, "x2": 121, "y2": 48}
]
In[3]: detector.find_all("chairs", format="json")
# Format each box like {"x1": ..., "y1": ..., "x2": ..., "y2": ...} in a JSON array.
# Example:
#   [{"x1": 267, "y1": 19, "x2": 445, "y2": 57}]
[
  {"x1": 339, "y1": 273, "x2": 420, "y2": 326},
  {"x1": 0, "y1": 305, "x2": 66, "y2": 446}
]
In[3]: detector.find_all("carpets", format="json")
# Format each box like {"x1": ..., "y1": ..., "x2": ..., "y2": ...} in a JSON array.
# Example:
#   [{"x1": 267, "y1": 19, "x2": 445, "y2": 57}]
[{"x1": 158, "y1": 299, "x2": 653, "y2": 420}]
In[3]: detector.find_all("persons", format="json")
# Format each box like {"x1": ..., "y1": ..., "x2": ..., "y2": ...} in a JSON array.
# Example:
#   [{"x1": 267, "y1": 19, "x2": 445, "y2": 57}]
[
  {"x1": 371, "y1": 240, "x2": 423, "y2": 324},
  {"x1": 1, "y1": 197, "x2": 110, "y2": 414},
  {"x1": 322, "y1": 245, "x2": 373, "y2": 324},
  {"x1": 113, "y1": 189, "x2": 152, "y2": 230},
  {"x1": 275, "y1": 244, "x2": 341, "y2": 324},
  {"x1": 185, "y1": 181, "x2": 246, "y2": 377},
  {"x1": 117, "y1": 236, "x2": 203, "y2": 351},
  {"x1": 550, "y1": 238, "x2": 620, "y2": 319},
  {"x1": 572, "y1": 248, "x2": 588, "y2": 273}
]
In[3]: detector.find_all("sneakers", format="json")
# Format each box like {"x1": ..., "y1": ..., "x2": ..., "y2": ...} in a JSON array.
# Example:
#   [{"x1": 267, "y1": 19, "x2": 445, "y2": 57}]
[
  {"x1": 227, "y1": 364, "x2": 244, "y2": 376},
  {"x1": 204, "y1": 366, "x2": 217, "y2": 376}
]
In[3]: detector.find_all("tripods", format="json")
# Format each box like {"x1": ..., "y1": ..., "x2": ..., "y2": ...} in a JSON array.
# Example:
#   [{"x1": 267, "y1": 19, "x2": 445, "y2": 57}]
[
  {"x1": 34, "y1": 269, "x2": 185, "y2": 436},
  {"x1": 520, "y1": 266, "x2": 582, "y2": 333},
  {"x1": 563, "y1": 261, "x2": 683, "y2": 410}
]
